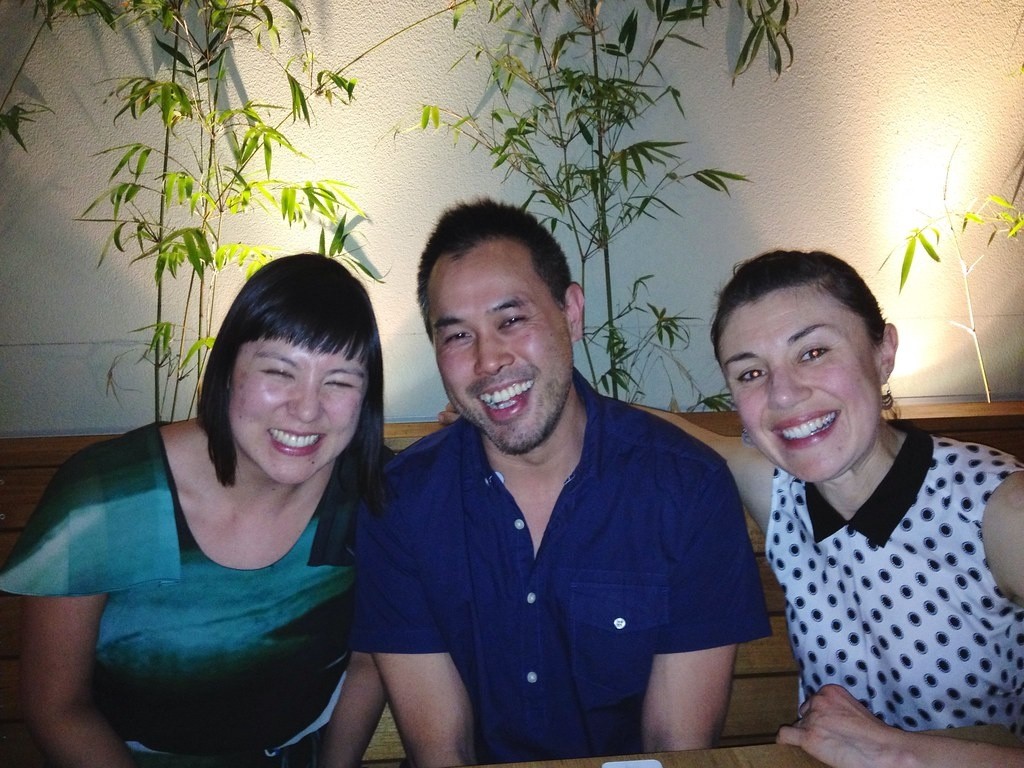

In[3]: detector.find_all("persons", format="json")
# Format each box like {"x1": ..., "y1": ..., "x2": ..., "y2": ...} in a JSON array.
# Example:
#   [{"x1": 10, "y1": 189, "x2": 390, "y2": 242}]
[
  {"x1": 435, "y1": 249, "x2": 1022, "y2": 768},
  {"x1": 346, "y1": 197, "x2": 774, "y2": 767},
  {"x1": 1, "y1": 252, "x2": 417, "y2": 767}
]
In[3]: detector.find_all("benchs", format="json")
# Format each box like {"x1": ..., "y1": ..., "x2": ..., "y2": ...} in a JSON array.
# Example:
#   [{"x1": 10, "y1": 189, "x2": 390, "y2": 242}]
[{"x1": 2, "y1": 405, "x2": 1024, "y2": 767}]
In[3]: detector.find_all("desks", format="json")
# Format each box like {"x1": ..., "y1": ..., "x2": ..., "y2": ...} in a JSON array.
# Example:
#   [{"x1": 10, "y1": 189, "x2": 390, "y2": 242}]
[{"x1": 485, "y1": 725, "x2": 1024, "y2": 768}]
